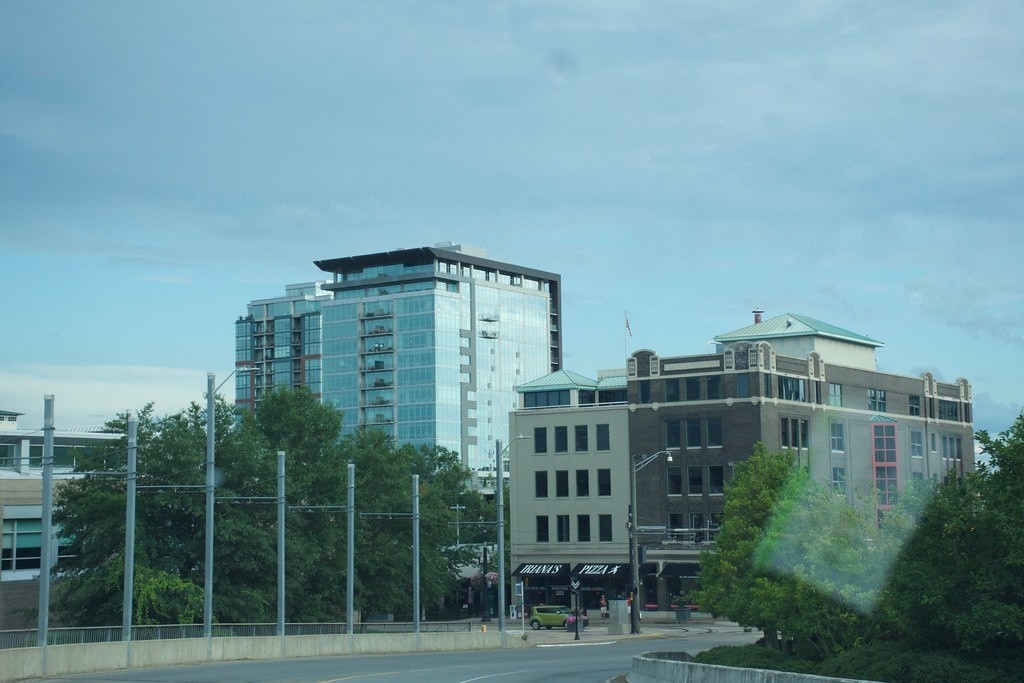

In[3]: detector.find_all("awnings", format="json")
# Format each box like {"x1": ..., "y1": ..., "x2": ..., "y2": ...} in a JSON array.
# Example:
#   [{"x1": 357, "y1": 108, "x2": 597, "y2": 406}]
[
  {"x1": 570, "y1": 563, "x2": 629, "y2": 579},
  {"x1": 511, "y1": 563, "x2": 570, "y2": 577}
]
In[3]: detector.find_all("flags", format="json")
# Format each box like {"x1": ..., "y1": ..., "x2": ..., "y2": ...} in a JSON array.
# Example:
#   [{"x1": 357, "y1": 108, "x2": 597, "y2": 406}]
[{"x1": 626, "y1": 319, "x2": 633, "y2": 336}]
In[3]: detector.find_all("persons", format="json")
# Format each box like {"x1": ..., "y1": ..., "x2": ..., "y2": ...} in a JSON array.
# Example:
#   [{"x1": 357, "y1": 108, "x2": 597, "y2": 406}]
[
  {"x1": 626, "y1": 596, "x2": 631, "y2": 614},
  {"x1": 600, "y1": 595, "x2": 606, "y2": 619}
]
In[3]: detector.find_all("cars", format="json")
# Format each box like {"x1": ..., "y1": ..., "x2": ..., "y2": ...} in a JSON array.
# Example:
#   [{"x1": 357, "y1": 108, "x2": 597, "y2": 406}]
[{"x1": 529, "y1": 605, "x2": 589, "y2": 630}]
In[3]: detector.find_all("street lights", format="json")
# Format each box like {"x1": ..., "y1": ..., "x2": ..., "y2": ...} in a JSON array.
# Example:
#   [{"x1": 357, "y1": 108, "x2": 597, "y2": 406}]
[
  {"x1": 631, "y1": 450, "x2": 674, "y2": 633},
  {"x1": 203, "y1": 364, "x2": 261, "y2": 637},
  {"x1": 496, "y1": 434, "x2": 532, "y2": 632}
]
